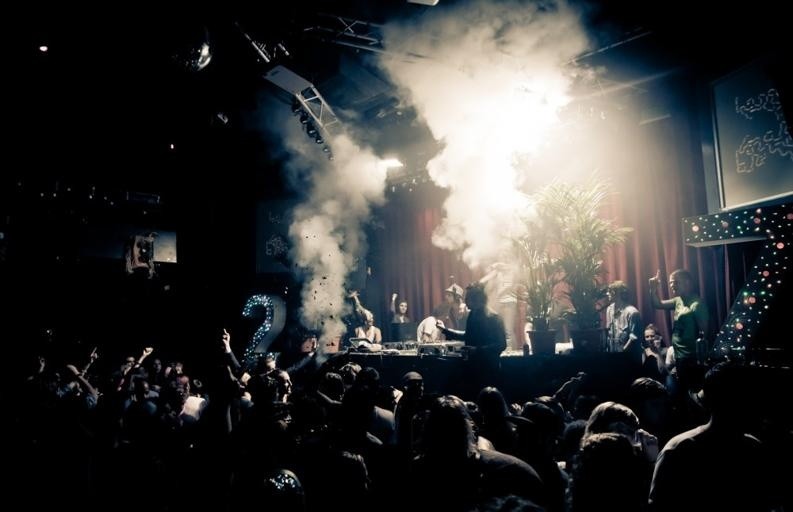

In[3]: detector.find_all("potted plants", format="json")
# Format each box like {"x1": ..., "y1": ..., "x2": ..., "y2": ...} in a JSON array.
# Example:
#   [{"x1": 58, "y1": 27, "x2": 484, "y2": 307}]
[
  {"x1": 506, "y1": 232, "x2": 566, "y2": 358},
  {"x1": 529, "y1": 176, "x2": 635, "y2": 358}
]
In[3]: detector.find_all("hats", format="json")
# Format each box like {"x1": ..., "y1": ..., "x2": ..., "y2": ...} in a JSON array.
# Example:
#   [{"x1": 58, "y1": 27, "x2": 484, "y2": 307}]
[{"x1": 444, "y1": 282, "x2": 464, "y2": 298}]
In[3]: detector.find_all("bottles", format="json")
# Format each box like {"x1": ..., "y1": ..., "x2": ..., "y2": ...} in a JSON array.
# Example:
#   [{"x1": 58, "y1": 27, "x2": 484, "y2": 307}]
[
  {"x1": 523, "y1": 343, "x2": 530, "y2": 356},
  {"x1": 311, "y1": 334, "x2": 317, "y2": 350}
]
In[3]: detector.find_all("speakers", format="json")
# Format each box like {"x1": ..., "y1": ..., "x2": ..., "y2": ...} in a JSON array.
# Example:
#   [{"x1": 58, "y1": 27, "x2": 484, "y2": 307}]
[{"x1": 151, "y1": 231, "x2": 178, "y2": 263}]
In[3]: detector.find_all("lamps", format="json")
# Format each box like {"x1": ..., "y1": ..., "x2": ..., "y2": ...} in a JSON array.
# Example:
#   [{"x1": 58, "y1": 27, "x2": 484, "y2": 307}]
[{"x1": 290, "y1": 98, "x2": 337, "y2": 162}]
